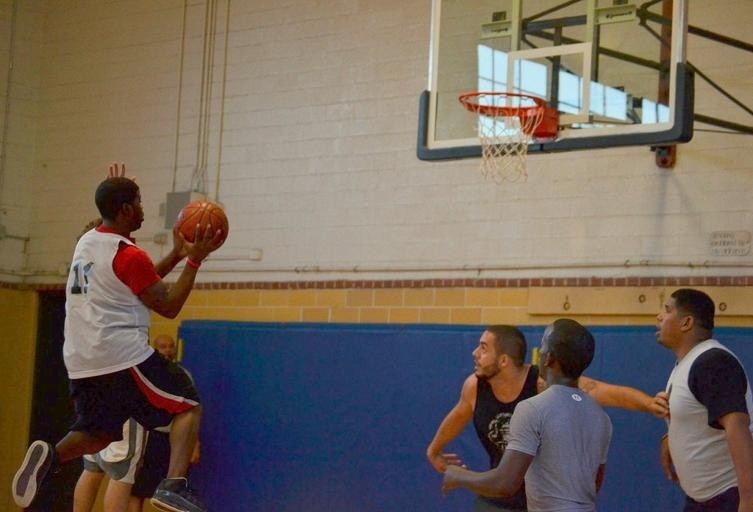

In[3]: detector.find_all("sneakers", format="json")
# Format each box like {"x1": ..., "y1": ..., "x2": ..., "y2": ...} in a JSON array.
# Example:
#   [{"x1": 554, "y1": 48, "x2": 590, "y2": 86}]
[
  {"x1": 12, "y1": 440, "x2": 55, "y2": 508},
  {"x1": 150, "y1": 477, "x2": 208, "y2": 512}
]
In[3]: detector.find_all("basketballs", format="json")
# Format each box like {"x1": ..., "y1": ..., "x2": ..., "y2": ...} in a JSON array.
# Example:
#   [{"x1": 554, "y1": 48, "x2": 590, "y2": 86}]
[{"x1": 176, "y1": 201, "x2": 228, "y2": 249}]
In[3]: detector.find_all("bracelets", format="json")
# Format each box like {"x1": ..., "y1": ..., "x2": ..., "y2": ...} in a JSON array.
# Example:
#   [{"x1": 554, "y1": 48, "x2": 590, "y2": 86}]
[{"x1": 186, "y1": 257, "x2": 203, "y2": 268}]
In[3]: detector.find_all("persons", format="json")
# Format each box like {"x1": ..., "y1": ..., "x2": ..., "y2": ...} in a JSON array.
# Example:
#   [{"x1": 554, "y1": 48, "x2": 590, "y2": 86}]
[
  {"x1": 654, "y1": 288, "x2": 753, "y2": 512},
  {"x1": 71, "y1": 161, "x2": 150, "y2": 512},
  {"x1": 426, "y1": 325, "x2": 671, "y2": 511},
  {"x1": 126, "y1": 333, "x2": 202, "y2": 512},
  {"x1": 441, "y1": 318, "x2": 614, "y2": 511},
  {"x1": 10, "y1": 177, "x2": 225, "y2": 512}
]
